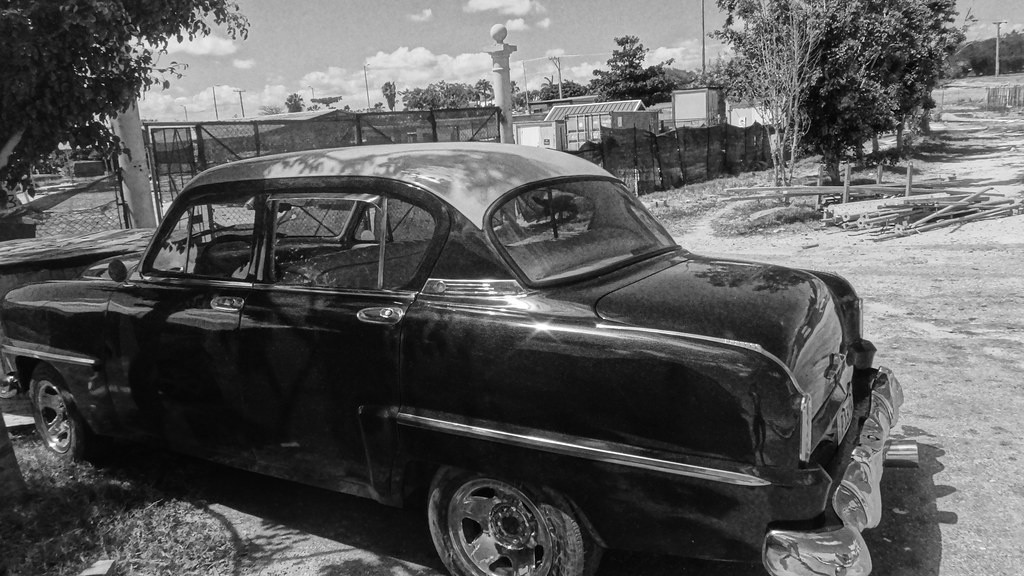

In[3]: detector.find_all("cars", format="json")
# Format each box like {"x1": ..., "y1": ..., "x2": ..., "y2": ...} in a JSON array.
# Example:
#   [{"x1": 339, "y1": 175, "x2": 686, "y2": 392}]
[{"x1": 0, "y1": 141, "x2": 906, "y2": 576}]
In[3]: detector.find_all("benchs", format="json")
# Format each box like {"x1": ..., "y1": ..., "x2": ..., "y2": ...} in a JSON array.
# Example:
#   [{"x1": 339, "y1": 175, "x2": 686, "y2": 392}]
[
  {"x1": 504, "y1": 224, "x2": 645, "y2": 280},
  {"x1": 282, "y1": 238, "x2": 431, "y2": 286}
]
